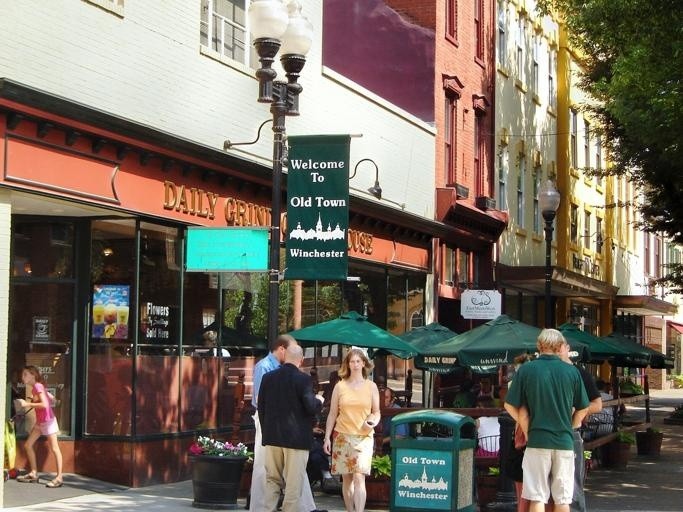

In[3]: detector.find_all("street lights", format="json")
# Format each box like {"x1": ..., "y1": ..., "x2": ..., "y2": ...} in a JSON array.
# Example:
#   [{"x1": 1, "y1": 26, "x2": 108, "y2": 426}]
[
  {"x1": 247, "y1": 0, "x2": 313, "y2": 507},
  {"x1": 537, "y1": 178, "x2": 562, "y2": 328}
]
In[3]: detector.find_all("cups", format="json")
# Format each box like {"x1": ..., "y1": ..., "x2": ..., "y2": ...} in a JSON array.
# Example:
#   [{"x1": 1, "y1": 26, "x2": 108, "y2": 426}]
[{"x1": 93, "y1": 305, "x2": 129, "y2": 326}]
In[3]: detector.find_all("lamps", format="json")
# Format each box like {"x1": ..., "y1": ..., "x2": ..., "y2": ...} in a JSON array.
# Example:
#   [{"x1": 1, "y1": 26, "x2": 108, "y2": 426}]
[
  {"x1": 581, "y1": 232, "x2": 615, "y2": 251},
  {"x1": 350, "y1": 158, "x2": 383, "y2": 200}
]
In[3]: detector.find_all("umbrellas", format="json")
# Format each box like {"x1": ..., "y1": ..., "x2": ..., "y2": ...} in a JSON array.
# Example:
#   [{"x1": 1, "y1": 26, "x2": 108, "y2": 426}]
[
  {"x1": 413, "y1": 312, "x2": 591, "y2": 376},
  {"x1": 556, "y1": 321, "x2": 632, "y2": 364},
  {"x1": 604, "y1": 332, "x2": 675, "y2": 378},
  {"x1": 374, "y1": 322, "x2": 460, "y2": 407},
  {"x1": 290, "y1": 309, "x2": 420, "y2": 360}
]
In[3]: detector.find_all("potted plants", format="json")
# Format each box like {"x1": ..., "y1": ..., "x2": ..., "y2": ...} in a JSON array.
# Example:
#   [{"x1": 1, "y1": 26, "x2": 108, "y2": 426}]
[
  {"x1": 613, "y1": 427, "x2": 663, "y2": 465},
  {"x1": 478, "y1": 467, "x2": 501, "y2": 508},
  {"x1": 365, "y1": 457, "x2": 392, "y2": 505}
]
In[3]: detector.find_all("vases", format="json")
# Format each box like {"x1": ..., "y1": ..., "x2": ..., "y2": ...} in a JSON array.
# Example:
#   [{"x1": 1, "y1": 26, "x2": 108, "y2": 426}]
[{"x1": 189, "y1": 455, "x2": 243, "y2": 506}]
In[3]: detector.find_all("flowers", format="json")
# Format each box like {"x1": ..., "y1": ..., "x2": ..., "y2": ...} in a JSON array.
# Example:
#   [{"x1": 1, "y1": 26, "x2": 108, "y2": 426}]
[{"x1": 189, "y1": 435, "x2": 252, "y2": 463}]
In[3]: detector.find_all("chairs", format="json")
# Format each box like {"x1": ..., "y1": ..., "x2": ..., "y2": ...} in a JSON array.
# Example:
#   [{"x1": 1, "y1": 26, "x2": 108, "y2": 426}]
[
  {"x1": 476, "y1": 436, "x2": 501, "y2": 458},
  {"x1": 586, "y1": 414, "x2": 615, "y2": 468}
]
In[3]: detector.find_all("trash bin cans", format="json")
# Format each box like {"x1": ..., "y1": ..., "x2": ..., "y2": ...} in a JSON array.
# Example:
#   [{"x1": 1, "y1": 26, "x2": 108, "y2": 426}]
[{"x1": 389, "y1": 409, "x2": 477, "y2": 512}]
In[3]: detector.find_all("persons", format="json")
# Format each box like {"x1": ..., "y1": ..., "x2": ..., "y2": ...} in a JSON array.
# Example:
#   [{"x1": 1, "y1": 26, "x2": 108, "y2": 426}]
[
  {"x1": 591, "y1": 380, "x2": 614, "y2": 434},
  {"x1": 504, "y1": 353, "x2": 552, "y2": 512},
  {"x1": 322, "y1": 347, "x2": 382, "y2": 511},
  {"x1": 373, "y1": 388, "x2": 410, "y2": 443},
  {"x1": 189, "y1": 330, "x2": 233, "y2": 358},
  {"x1": 558, "y1": 336, "x2": 605, "y2": 512},
  {"x1": 327, "y1": 371, "x2": 342, "y2": 402},
  {"x1": 248, "y1": 334, "x2": 328, "y2": 511},
  {"x1": 452, "y1": 376, "x2": 505, "y2": 456},
  {"x1": 256, "y1": 345, "x2": 327, "y2": 511},
  {"x1": 16, "y1": 364, "x2": 64, "y2": 488},
  {"x1": 503, "y1": 328, "x2": 592, "y2": 512}
]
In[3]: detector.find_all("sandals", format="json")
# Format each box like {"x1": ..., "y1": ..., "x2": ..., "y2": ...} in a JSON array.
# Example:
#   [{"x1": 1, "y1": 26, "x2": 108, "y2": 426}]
[
  {"x1": 46, "y1": 479, "x2": 64, "y2": 488},
  {"x1": 17, "y1": 473, "x2": 39, "y2": 483}
]
